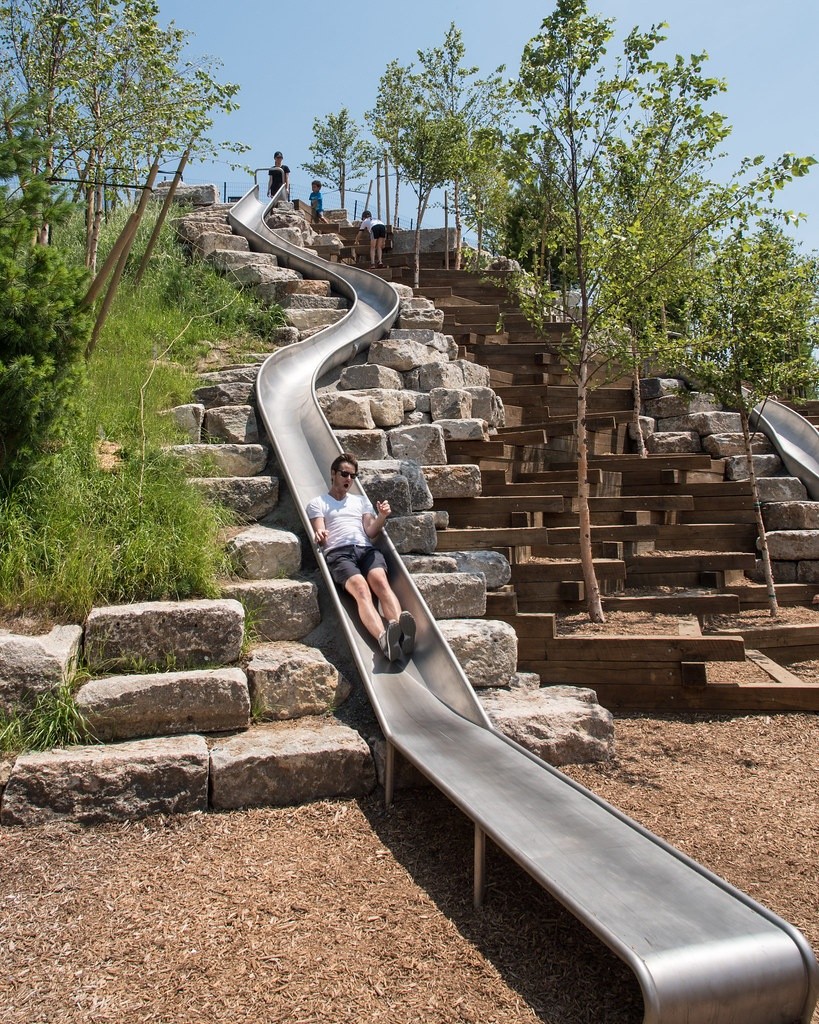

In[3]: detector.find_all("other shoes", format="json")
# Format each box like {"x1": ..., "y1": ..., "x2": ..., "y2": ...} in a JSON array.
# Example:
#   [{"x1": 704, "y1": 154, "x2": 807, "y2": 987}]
[
  {"x1": 398, "y1": 610, "x2": 417, "y2": 655},
  {"x1": 378, "y1": 264, "x2": 383, "y2": 268},
  {"x1": 382, "y1": 620, "x2": 402, "y2": 663},
  {"x1": 370, "y1": 262, "x2": 376, "y2": 268}
]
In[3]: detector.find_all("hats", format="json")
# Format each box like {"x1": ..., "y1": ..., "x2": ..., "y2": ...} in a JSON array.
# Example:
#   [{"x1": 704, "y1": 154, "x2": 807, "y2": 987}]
[{"x1": 361, "y1": 211, "x2": 371, "y2": 220}]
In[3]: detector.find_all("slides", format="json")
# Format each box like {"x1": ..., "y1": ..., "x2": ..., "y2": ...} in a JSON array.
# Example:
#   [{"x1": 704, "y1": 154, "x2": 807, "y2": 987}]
[
  {"x1": 738, "y1": 384, "x2": 819, "y2": 498},
  {"x1": 226, "y1": 178, "x2": 819, "y2": 1024}
]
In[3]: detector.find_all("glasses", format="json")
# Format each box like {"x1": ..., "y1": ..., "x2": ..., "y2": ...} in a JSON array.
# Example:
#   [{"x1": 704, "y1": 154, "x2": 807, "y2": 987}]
[{"x1": 336, "y1": 469, "x2": 359, "y2": 479}]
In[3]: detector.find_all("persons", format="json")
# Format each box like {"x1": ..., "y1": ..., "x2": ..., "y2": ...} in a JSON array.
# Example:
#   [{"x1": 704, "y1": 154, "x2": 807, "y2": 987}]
[
  {"x1": 353, "y1": 210, "x2": 386, "y2": 269},
  {"x1": 266, "y1": 151, "x2": 291, "y2": 201},
  {"x1": 304, "y1": 452, "x2": 417, "y2": 665},
  {"x1": 308, "y1": 179, "x2": 330, "y2": 225}
]
